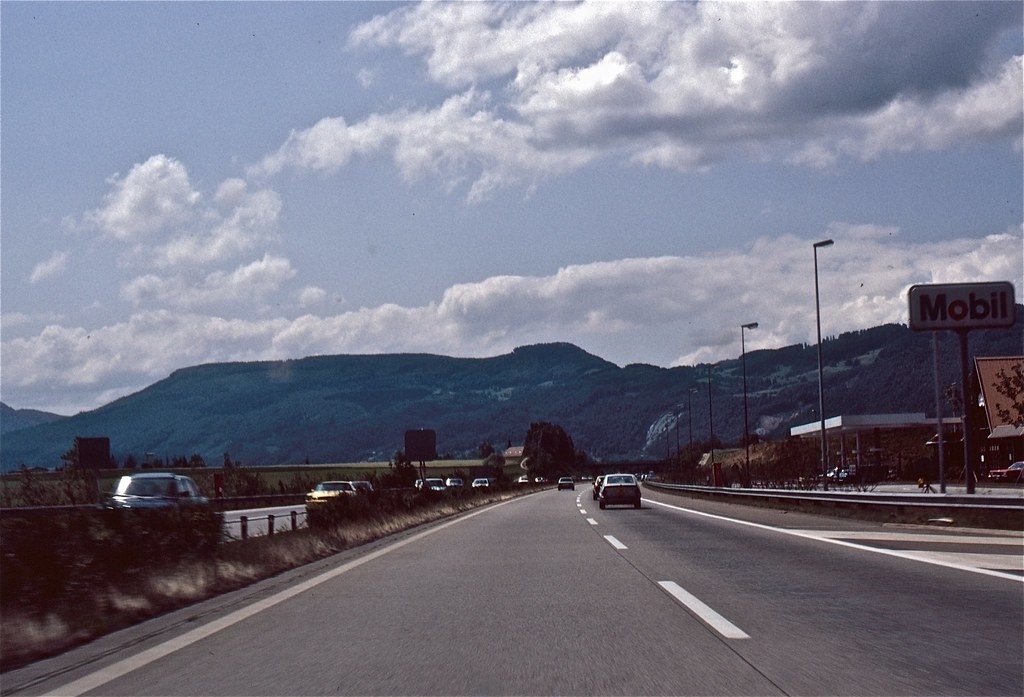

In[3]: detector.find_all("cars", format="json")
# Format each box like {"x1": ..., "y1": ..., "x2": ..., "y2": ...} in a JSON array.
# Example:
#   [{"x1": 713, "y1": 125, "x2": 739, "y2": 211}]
[
  {"x1": 415, "y1": 478, "x2": 447, "y2": 491},
  {"x1": 304, "y1": 480, "x2": 358, "y2": 504},
  {"x1": 518, "y1": 476, "x2": 547, "y2": 483},
  {"x1": 599, "y1": 473, "x2": 642, "y2": 509},
  {"x1": 351, "y1": 482, "x2": 373, "y2": 493},
  {"x1": 635, "y1": 472, "x2": 653, "y2": 481},
  {"x1": 108, "y1": 472, "x2": 207, "y2": 511},
  {"x1": 591, "y1": 476, "x2": 604, "y2": 500},
  {"x1": 446, "y1": 478, "x2": 463, "y2": 487},
  {"x1": 987, "y1": 461, "x2": 1024, "y2": 484},
  {"x1": 471, "y1": 478, "x2": 490, "y2": 487},
  {"x1": 556, "y1": 477, "x2": 575, "y2": 491}
]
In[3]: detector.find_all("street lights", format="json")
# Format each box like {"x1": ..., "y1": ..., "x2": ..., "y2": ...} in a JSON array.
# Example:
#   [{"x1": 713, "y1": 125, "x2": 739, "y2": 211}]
[
  {"x1": 813, "y1": 239, "x2": 835, "y2": 491},
  {"x1": 687, "y1": 387, "x2": 697, "y2": 483},
  {"x1": 708, "y1": 362, "x2": 722, "y2": 462},
  {"x1": 742, "y1": 321, "x2": 759, "y2": 487}
]
